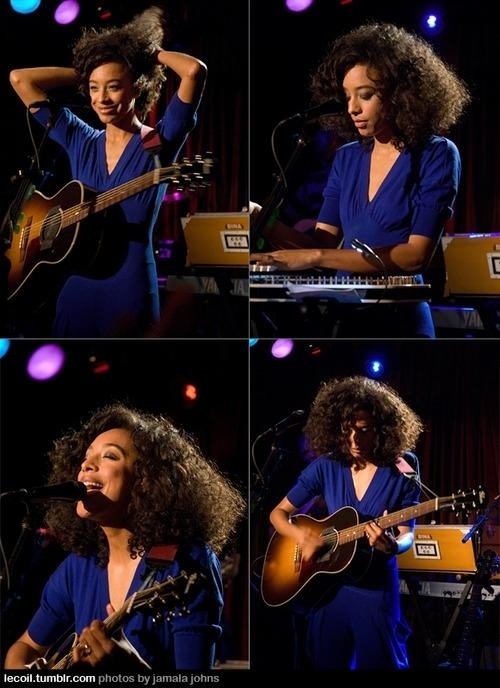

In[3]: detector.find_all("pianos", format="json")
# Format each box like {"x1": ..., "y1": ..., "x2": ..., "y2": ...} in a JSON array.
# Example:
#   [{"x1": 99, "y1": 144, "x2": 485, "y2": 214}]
[
  {"x1": 248, "y1": 263, "x2": 433, "y2": 299},
  {"x1": 400, "y1": 579, "x2": 500, "y2": 601}
]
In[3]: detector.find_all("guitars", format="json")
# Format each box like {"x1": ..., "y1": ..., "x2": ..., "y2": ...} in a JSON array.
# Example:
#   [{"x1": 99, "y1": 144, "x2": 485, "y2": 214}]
[
  {"x1": 261, "y1": 487, "x2": 487, "y2": 616},
  {"x1": 44, "y1": 570, "x2": 205, "y2": 670},
  {"x1": 5, "y1": 152, "x2": 215, "y2": 306}
]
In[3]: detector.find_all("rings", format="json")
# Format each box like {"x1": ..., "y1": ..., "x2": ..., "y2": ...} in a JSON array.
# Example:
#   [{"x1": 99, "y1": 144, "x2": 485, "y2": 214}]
[
  {"x1": 366, "y1": 531, "x2": 370, "y2": 536},
  {"x1": 85, "y1": 643, "x2": 91, "y2": 652}
]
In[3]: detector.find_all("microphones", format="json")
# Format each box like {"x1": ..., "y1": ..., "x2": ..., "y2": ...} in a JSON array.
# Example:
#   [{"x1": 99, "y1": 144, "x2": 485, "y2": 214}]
[
  {"x1": 275, "y1": 407, "x2": 304, "y2": 432},
  {"x1": 7, "y1": 479, "x2": 89, "y2": 499},
  {"x1": 29, "y1": 95, "x2": 92, "y2": 110},
  {"x1": 298, "y1": 95, "x2": 347, "y2": 120}
]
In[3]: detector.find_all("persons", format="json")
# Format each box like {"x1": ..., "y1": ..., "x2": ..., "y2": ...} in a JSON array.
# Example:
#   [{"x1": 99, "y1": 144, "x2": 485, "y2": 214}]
[
  {"x1": 269, "y1": 377, "x2": 426, "y2": 667},
  {"x1": 3, "y1": 406, "x2": 245, "y2": 670},
  {"x1": 250, "y1": 18, "x2": 472, "y2": 339},
  {"x1": 7, "y1": 6, "x2": 208, "y2": 337}
]
